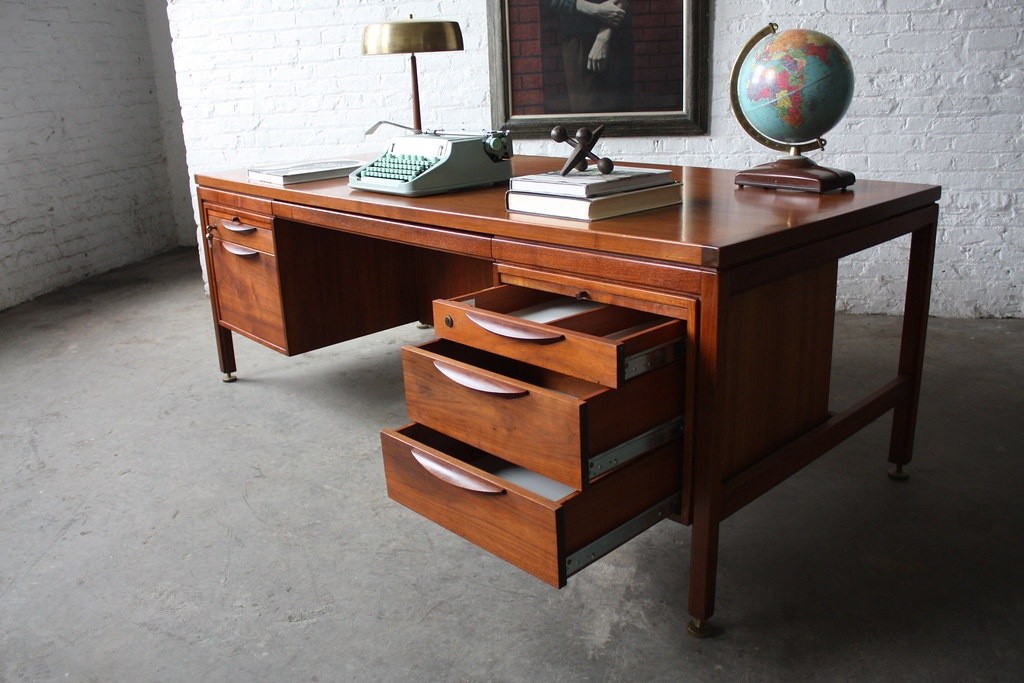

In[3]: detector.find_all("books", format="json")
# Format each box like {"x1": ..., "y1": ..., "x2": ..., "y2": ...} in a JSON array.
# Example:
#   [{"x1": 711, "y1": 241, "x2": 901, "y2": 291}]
[
  {"x1": 504, "y1": 162, "x2": 684, "y2": 222},
  {"x1": 247, "y1": 160, "x2": 362, "y2": 185}
]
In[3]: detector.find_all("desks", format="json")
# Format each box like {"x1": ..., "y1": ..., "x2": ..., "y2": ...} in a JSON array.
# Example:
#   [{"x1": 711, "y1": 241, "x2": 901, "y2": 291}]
[{"x1": 195, "y1": 153, "x2": 941, "y2": 639}]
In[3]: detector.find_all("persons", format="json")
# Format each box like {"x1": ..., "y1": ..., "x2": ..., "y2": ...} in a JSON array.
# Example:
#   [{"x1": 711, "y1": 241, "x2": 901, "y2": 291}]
[{"x1": 550, "y1": 0, "x2": 627, "y2": 113}]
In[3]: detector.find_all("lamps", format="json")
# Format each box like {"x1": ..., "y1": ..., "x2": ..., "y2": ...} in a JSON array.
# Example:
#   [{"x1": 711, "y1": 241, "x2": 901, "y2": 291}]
[{"x1": 362, "y1": 14, "x2": 464, "y2": 134}]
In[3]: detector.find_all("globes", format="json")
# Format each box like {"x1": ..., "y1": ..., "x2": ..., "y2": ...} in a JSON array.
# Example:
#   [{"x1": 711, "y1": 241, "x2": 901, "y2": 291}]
[{"x1": 728, "y1": 22, "x2": 856, "y2": 195}]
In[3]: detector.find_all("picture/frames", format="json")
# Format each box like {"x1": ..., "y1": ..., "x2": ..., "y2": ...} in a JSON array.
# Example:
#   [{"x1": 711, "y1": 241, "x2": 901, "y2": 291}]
[{"x1": 486, "y1": 0, "x2": 711, "y2": 140}]
